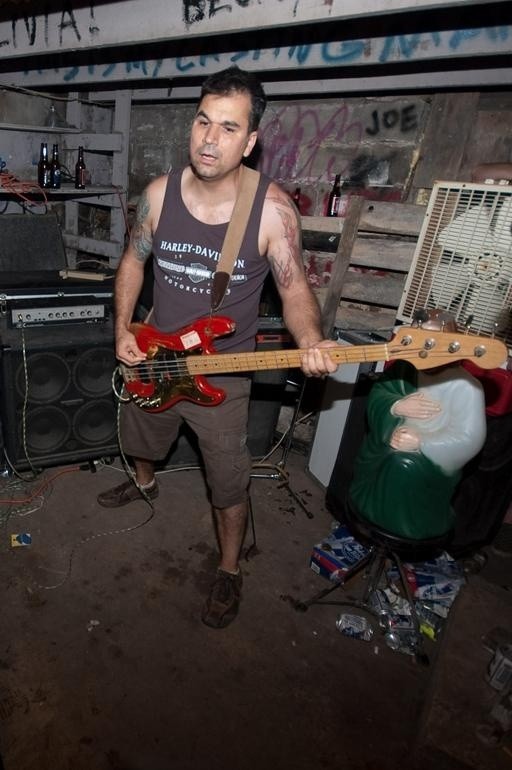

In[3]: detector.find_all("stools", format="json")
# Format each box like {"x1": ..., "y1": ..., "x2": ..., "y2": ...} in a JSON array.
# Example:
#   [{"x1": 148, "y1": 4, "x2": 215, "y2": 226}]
[{"x1": 299, "y1": 494, "x2": 458, "y2": 668}]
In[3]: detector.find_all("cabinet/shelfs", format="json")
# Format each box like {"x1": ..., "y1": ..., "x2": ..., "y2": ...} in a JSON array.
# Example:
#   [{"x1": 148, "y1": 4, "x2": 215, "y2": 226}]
[{"x1": 0, "y1": 94, "x2": 132, "y2": 277}]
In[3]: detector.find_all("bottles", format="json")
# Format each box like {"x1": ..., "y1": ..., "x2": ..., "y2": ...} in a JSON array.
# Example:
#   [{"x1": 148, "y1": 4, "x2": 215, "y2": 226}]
[
  {"x1": 294, "y1": 188, "x2": 301, "y2": 212},
  {"x1": 38, "y1": 142, "x2": 87, "y2": 190},
  {"x1": 327, "y1": 174, "x2": 341, "y2": 217}
]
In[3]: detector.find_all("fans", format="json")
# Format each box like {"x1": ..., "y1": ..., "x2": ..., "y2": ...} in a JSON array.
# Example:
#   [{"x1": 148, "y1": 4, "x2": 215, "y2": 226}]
[{"x1": 397, "y1": 180, "x2": 511, "y2": 350}]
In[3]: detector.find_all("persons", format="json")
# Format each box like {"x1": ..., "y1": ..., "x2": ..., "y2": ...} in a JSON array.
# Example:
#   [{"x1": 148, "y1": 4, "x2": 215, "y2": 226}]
[
  {"x1": 346, "y1": 305, "x2": 488, "y2": 543},
  {"x1": 93, "y1": 64, "x2": 344, "y2": 632}
]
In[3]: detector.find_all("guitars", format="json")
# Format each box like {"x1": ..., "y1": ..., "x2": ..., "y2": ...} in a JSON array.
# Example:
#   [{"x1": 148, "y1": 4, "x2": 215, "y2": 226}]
[{"x1": 120, "y1": 314, "x2": 508, "y2": 413}]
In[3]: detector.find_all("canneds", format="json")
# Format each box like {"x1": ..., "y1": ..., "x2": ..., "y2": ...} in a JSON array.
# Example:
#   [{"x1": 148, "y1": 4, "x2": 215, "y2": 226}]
[
  {"x1": 337, "y1": 613, "x2": 373, "y2": 643},
  {"x1": 378, "y1": 609, "x2": 400, "y2": 653}
]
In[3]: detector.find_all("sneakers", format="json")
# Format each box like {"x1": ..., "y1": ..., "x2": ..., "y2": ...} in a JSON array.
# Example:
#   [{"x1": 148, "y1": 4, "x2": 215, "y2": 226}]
[
  {"x1": 97, "y1": 478, "x2": 159, "y2": 508},
  {"x1": 203, "y1": 568, "x2": 243, "y2": 628}
]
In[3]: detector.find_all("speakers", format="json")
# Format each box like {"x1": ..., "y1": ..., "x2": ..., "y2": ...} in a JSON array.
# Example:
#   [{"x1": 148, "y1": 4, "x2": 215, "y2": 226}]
[
  {"x1": 2, "y1": 296, "x2": 121, "y2": 472},
  {"x1": 164, "y1": 326, "x2": 297, "y2": 466}
]
